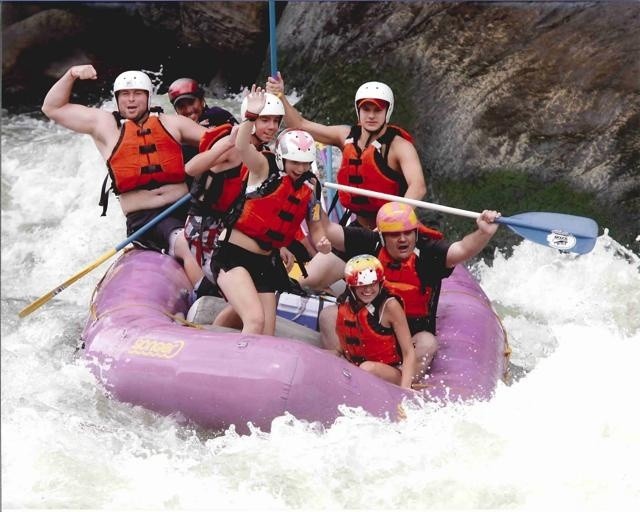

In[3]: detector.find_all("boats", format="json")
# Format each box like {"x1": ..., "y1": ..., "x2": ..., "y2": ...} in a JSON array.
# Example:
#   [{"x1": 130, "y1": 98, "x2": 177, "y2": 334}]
[{"x1": 81, "y1": 171, "x2": 509, "y2": 441}]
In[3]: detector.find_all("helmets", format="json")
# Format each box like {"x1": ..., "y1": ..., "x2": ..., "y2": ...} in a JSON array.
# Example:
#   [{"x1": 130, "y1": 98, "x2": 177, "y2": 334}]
[
  {"x1": 343, "y1": 253, "x2": 385, "y2": 302},
  {"x1": 273, "y1": 127, "x2": 316, "y2": 173},
  {"x1": 111, "y1": 69, "x2": 153, "y2": 115},
  {"x1": 168, "y1": 76, "x2": 204, "y2": 107},
  {"x1": 240, "y1": 91, "x2": 286, "y2": 136},
  {"x1": 355, "y1": 81, "x2": 396, "y2": 124},
  {"x1": 375, "y1": 201, "x2": 419, "y2": 249}
]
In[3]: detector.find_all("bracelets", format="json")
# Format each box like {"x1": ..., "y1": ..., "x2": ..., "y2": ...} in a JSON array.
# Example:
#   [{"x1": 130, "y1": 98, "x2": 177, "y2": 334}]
[{"x1": 336, "y1": 349, "x2": 342, "y2": 354}]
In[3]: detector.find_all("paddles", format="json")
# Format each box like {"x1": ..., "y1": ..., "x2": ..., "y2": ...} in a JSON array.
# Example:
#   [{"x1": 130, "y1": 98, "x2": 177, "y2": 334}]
[
  {"x1": 18, "y1": 193, "x2": 194, "y2": 320},
  {"x1": 322, "y1": 182, "x2": 599, "y2": 256}
]
